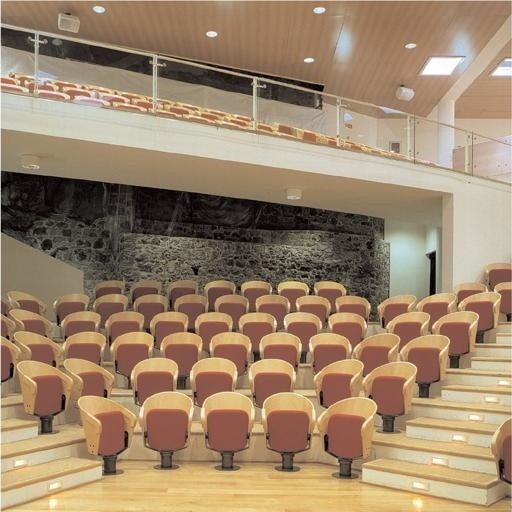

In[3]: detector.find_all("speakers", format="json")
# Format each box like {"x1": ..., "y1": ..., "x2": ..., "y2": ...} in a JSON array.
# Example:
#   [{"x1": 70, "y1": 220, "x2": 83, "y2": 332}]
[
  {"x1": 58, "y1": 13, "x2": 80, "y2": 33},
  {"x1": 396, "y1": 87, "x2": 415, "y2": 102}
]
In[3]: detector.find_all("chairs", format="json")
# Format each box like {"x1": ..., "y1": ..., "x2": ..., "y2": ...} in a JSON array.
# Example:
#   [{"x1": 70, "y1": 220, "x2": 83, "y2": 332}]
[
  {"x1": 139, "y1": 391, "x2": 195, "y2": 471},
  {"x1": 200, "y1": 391, "x2": 256, "y2": 471},
  {"x1": 77, "y1": 395, "x2": 137, "y2": 476},
  {"x1": 317, "y1": 397, "x2": 378, "y2": 478},
  {"x1": 261, "y1": 392, "x2": 316, "y2": 471},
  {"x1": 491, "y1": 416, "x2": 511, "y2": 484}
]
